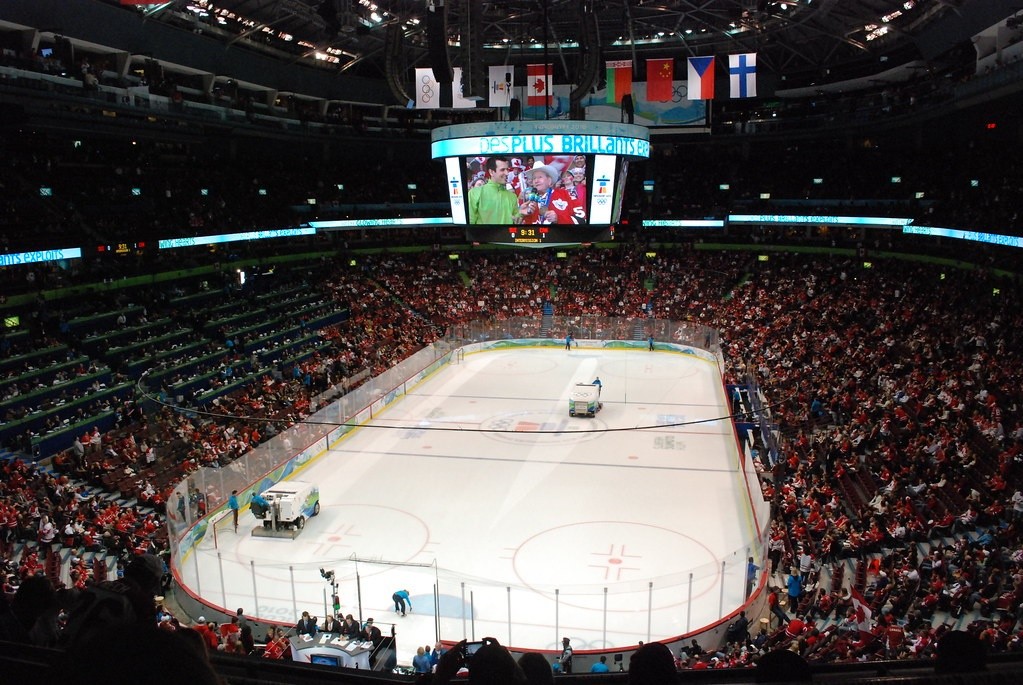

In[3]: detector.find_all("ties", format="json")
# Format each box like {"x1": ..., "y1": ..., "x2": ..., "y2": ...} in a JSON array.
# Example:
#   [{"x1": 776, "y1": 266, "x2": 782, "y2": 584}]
[
  {"x1": 368, "y1": 632, "x2": 371, "y2": 638},
  {"x1": 329, "y1": 623, "x2": 331, "y2": 631}
]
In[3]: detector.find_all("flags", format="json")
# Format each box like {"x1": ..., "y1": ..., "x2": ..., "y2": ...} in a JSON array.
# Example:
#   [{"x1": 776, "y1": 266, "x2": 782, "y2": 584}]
[
  {"x1": 527, "y1": 64, "x2": 553, "y2": 106},
  {"x1": 646, "y1": 58, "x2": 673, "y2": 102},
  {"x1": 415, "y1": 65, "x2": 514, "y2": 109},
  {"x1": 728, "y1": 53, "x2": 757, "y2": 98},
  {"x1": 687, "y1": 56, "x2": 715, "y2": 100},
  {"x1": 605, "y1": 60, "x2": 633, "y2": 104}
]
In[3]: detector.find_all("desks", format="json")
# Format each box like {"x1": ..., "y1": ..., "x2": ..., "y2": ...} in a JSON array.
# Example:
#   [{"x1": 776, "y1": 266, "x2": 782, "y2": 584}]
[{"x1": 0, "y1": 264, "x2": 352, "y2": 466}]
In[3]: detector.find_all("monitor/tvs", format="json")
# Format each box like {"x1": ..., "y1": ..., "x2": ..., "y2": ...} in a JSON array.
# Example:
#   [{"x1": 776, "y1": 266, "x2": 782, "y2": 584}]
[{"x1": 310, "y1": 654, "x2": 340, "y2": 667}]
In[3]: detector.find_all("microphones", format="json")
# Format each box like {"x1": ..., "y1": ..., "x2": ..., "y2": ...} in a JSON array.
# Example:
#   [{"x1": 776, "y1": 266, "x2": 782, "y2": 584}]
[{"x1": 527, "y1": 188, "x2": 539, "y2": 214}]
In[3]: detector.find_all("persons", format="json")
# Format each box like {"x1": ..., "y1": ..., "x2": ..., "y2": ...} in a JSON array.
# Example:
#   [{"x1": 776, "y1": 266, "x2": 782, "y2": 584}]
[
  {"x1": 176, "y1": 492, "x2": 186, "y2": 522},
  {"x1": 466, "y1": 154, "x2": 586, "y2": 224},
  {"x1": 0, "y1": 244, "x2": 1023, "y2": 685},
  {"x1": 194, "y1": 488, "x2": 204, "y2": 504},
  {"x1": 228, "y1": 489, "x2": 239, "y2": 528},
  {"x1": 648, "y1": 336, "x2": 654, "y2": 351},
  {"x1": 197, "y1": 498, "x2": 206, "y2": 519},
  {"x1": 251, "y1": 492, "x2": 271, "y2": 514},
  {"x1": 566, "y1": 334, "x2": 571, "y2": 350},
  {"x1": 392, "y1": 589, "x2": 412, "y2": 616},
  {"x1": 592, "y1": 377, "x2": 602, "y2": 397}
]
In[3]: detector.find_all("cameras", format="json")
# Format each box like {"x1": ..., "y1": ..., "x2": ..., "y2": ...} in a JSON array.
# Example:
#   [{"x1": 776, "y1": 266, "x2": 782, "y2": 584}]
[{"x1": 463, "y1": 641, "x2": 487, "y2": 658}]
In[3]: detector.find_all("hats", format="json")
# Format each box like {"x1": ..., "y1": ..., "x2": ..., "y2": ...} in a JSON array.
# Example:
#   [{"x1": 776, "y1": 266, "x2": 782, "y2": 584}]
[
  {"x1": 524, "y1": 161, "x2": 558, "y2": 186},
  {"x1": 567, "y1": 170, "x2": 574, "y2": 177},
  {"x1": 511, "y1": 158, "x2": 521, "y2": 168},
  {"x1": 199, "y1": 616, "x2": 205, "y2": 622}
]
